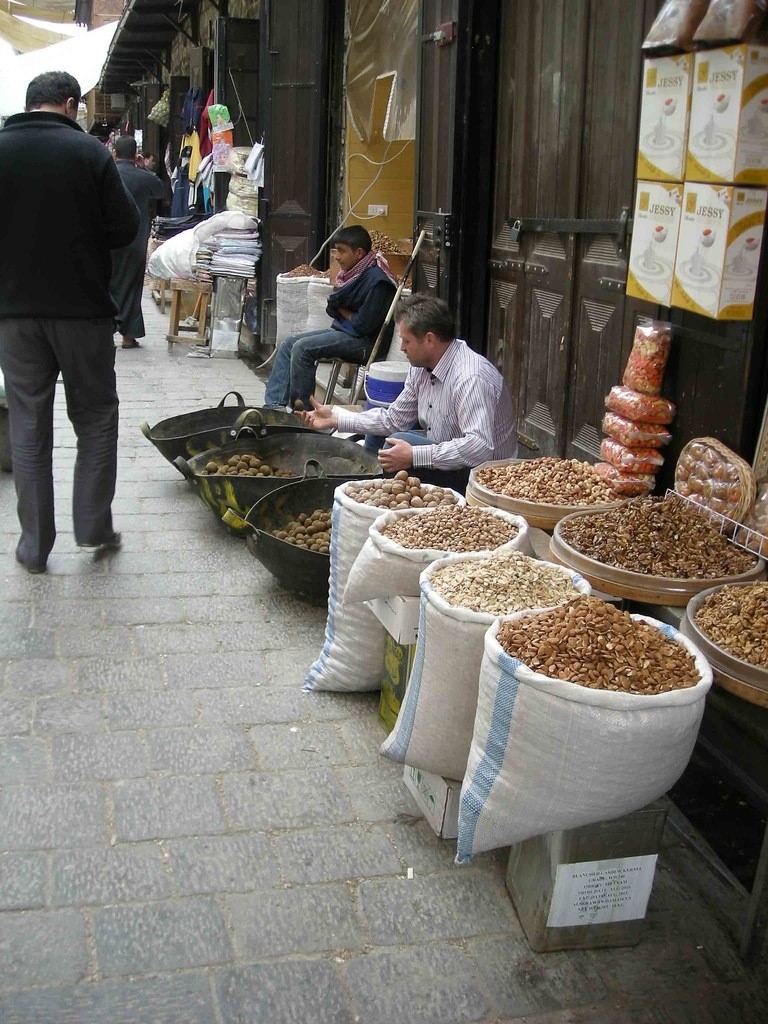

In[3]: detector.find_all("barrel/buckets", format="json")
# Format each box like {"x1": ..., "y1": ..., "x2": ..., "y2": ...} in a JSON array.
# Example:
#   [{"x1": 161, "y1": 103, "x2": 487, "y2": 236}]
[{"x1": 363, "y1": 361, "x2": 409, "y2": 411}]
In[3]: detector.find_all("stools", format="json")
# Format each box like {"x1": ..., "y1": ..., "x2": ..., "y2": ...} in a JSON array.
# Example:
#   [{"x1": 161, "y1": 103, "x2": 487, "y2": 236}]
[
  {"x1": 314, "y1": 357, "x2": 362, "y2": 405},
  {"x1": 165, "y1": 277, "x2": 213, "y2": 354}
]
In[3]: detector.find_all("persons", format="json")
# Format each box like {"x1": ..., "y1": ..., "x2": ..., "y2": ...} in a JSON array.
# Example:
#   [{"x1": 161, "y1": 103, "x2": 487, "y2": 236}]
[
  {"x1": 263, "y1": 225, "x2": 396, "y2": 411},
  {"x1": 0, "y1": 69, "x2": 142, "y2": 573},
  {"x1": 106, "y1": 136, "x2": 170, "y2": 348},
  {"x1": 294, "y1": 291, "x2": 518, "y2": 496}
]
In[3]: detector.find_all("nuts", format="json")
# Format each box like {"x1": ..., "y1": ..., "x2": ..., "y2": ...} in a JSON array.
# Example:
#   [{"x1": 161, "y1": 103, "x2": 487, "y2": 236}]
[
  {"x1": 295, "y1": 399, "x2": 304, "y2": 408},
  {"x1": 272, "y1": 508, "x2": 333, "y2": 554},
  {"x1": 345, "y1": 471, "x2": 458, "y2": 511},
  {"x1": 559, "y1": 496, "x2": 758, "y2": 580},
  {"x1": 496, "y1": 594, "x2": 702, "y2": 696},
  {"x1": 696, "y1": 580, "x2": 768, "y2": 670},
  {"x1": 379, "y1": 503, "x2": 520, "y2": 553},
  {"x1": 476, "y1": 454, "x2": 625, "y2": 505},
  {"x1": 200, "y1": 454, "x2": 300, "y2": 478}
]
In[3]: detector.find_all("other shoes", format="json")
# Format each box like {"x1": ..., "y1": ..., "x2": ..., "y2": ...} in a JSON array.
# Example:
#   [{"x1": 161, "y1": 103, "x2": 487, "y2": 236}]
[
  {"x1": 14, "y1": 551, "x2": 45, "y2": 573},
  {"x1": 273, "y1": 404, "x2": 287, "y2": 414},
  {"x1": 78, "y1": 530, "x2": 120, "y2": 547}
]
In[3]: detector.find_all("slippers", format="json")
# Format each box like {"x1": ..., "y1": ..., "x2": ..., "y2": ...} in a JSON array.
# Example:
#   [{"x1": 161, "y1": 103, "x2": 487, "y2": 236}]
[{"x1": 122, "y1": 338, "x2": 140, "y2": 348}]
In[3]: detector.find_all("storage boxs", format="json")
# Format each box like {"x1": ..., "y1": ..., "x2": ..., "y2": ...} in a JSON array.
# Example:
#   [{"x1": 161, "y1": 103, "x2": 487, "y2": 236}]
[
  {"x1": 685, "y1": 42, "x2": 768, "y2": 188},
  {"x1": 377, "y1": 631, "x2": 415, "y2": 735},
  {"x1": 670, "y1": 182, "x2": 768, "y2": 322},
  {"x1": 625, "y1": 179, "x2": 683, "y2": 308},
  {"x1": 363, "y1": 595, "x2": 420, "y2": 646},
  {"x1": 637, "y1": 54, "x2": 695, "y2": 183},
  {"x1": 505, "y1": 796, "x2": 670, "y2": 954},
  {"x1": 403, "y1": 764, "x2": 463, "y2": 839}
]
